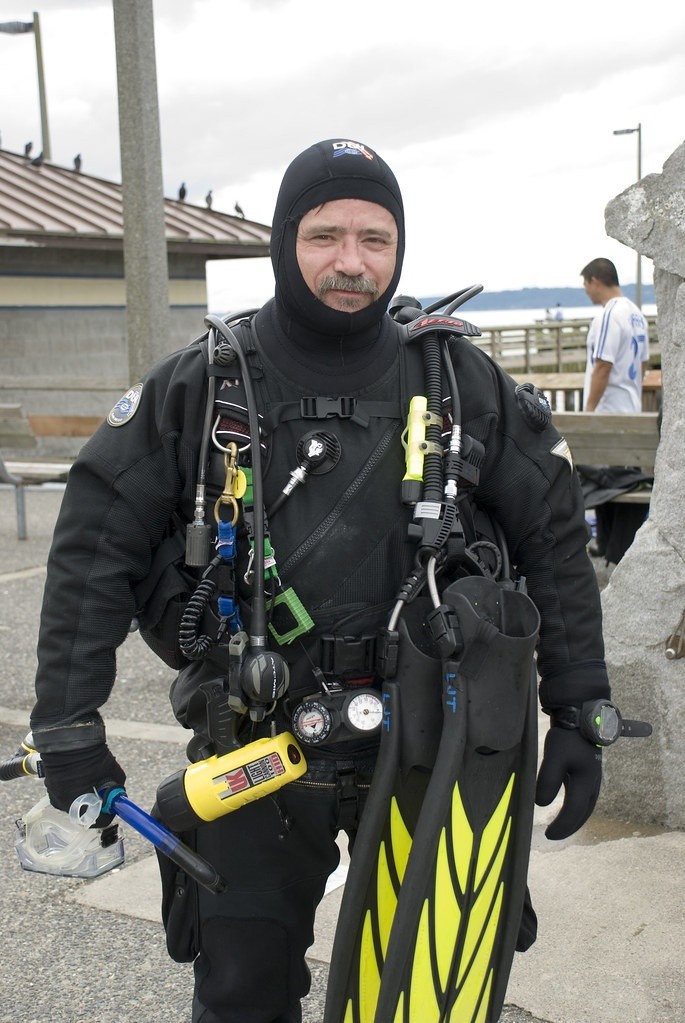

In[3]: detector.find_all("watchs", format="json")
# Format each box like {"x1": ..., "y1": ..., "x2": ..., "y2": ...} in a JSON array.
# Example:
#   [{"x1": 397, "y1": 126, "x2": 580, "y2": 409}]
[{"x1": 548, "y1": 699, "x2": 653, "y2": 748}]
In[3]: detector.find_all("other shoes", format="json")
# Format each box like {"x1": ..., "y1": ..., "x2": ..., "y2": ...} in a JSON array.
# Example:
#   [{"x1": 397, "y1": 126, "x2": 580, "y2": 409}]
[{"x1": 589, "y1": 545, "x2": 604, "y2": 558}]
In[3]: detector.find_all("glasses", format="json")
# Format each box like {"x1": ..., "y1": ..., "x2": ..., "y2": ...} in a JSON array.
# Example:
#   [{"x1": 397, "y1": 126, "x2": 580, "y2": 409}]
[{"x1": 14, "y1": 799, "x2": 125, "y2": 879}]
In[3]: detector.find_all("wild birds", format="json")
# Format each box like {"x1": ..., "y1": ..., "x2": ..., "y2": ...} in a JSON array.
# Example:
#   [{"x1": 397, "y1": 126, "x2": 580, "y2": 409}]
[
  {"x1": 31, "y1": 152, "x2": 46, "y2": 167},
  {"x1": 235, "y1": 202, "x2": 245, "y2": 217},
  {"x1": 25, "y1": 142, "x2": 32, "y2": 157},
  {"x1": 206, "y1": 190, "x2": 213, "y2": 209},
  {"x1": 179, "y1": 183, "x2": 186, "y2": 200},
  {"x1": 74, "y1": 154, "x2": 81, "y2": 171}
]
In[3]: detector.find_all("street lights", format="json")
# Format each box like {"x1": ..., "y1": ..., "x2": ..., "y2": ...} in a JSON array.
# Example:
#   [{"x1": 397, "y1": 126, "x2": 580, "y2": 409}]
[
  {"x1": 0, "y1": 12, "x2": 52, "y2": 164},
  {"x1": 611, "y1": 121, "x2": 642, "y2": 313}
]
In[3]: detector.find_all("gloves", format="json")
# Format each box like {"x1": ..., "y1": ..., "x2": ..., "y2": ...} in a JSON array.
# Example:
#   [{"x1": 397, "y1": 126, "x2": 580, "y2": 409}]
[
  {"x1": 535, "y1": 726, "x2": 604, "y2": 840},
  {"x1": 41, "y1": 743, "x2": 126, "y2": 828}
]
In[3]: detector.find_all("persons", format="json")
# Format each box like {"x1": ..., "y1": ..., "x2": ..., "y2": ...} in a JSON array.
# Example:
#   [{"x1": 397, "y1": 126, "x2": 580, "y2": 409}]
[
  {"x1": 31, "y1": 138, "x2": 653, "y2": 1023},
  {"x1": 581, "y1": 258, "x2": 650, "y2": 555}
]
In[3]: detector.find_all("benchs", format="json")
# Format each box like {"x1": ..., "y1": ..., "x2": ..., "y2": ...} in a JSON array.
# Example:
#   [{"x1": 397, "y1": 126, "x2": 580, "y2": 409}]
[
  {"x1": 551, "y1": 409, "x2": 660, "y2": 503},
  {"x1": 0, "y1": 403, "x2": 74, "y2": 540}
]
